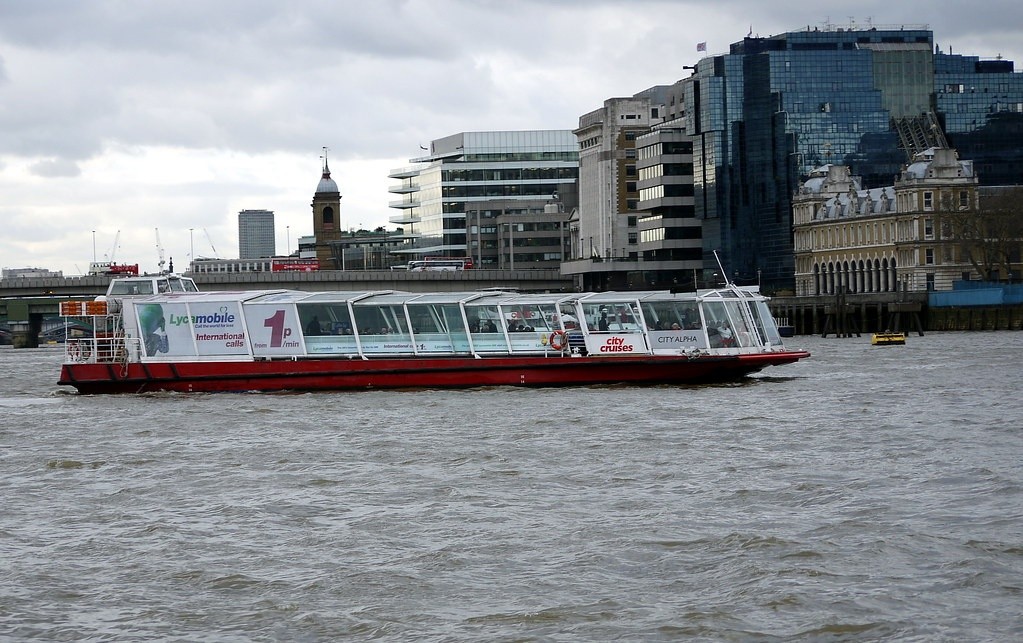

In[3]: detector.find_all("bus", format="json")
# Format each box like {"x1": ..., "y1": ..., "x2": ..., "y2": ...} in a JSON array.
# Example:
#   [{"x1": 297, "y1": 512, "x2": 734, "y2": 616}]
[
  {"x1": 408, "y1": 260, "x2": 465, "y2": 273},
  {"x1": 271, "y1": 258, "x2": 320, "y2": 273},
  {"x1": 424, "y1": 257, "x2": 474, "y2": 269}
]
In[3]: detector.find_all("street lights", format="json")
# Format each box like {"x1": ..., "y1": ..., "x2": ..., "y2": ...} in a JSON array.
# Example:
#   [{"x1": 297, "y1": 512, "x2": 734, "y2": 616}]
[
  {"x1": 188, "y1": 228, "x2": 194, "y2": 265},
  {"x1": 92, "y1": 230, "x2": 97, "y2": 263},
  {"x1": 287, "y1": 225, "x2": 289, "y2": 255}
]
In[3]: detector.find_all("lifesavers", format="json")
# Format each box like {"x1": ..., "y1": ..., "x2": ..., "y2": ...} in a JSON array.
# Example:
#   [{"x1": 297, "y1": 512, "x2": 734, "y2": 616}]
[
  {"x1": 550, "y1": 330, "x2": 568, "y2": 350},
  {"x1": 67, "y1": 343, "x2": 81, "y2": 357}
]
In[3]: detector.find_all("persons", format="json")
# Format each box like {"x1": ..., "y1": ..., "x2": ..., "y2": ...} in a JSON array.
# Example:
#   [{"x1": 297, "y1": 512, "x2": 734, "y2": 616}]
[
  {"x1": 573, "y1": 323, "x2": 581, "y2": 330},
  {"x1": 306, "y1": 313, "x2": 321, "y2": 336},
  {"x1": 345, "y1": 326, "x2": 394, "y2": 335},
  {"x1": 469, "y1": 319, "x2": 498, "y2": 333},
  {"x1": 598, "y1": 312, "x2": 611, "y2": 334},
  {"x1": 413, "y1": 328, "x2": 419, "y2": 334},
  {"x1": 652, "y1": 321, "x2": 702, "y2": 330},
  {"x1": 506, "y1": 319, "x2": 536, "y2": 332},
  {"x1": 706, "y1": 319, "x2": 746, "y2": 348},
  {"x1": 587, "y1": 323, "x2": 596, "y2": 331}
]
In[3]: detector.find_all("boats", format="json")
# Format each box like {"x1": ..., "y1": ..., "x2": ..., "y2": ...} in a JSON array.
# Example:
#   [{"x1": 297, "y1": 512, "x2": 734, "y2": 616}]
[
  {"x1": 54, "y1": 275, "x2": 816, "y2": 400},
  {"x1": 871, "y1": 332, "x2": 906, "y2": 345}
]
